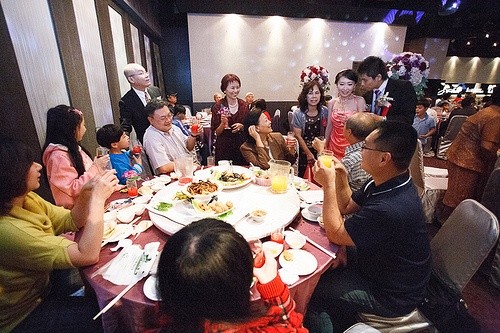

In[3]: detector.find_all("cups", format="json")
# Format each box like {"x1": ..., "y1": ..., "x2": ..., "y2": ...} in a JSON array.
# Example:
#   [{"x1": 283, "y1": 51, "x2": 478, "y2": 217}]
[
  {"x1": 96, "y1": 147, "x2": 107, "y2": 156},
  {"x1": 126, "y1": 179, "x2": 138, "y2": 198},
  {"x1": 132, "y1": 139, "x2": 141, "y2": 154},
  {"x1": 271, "y1": 226, "x2": 284, "y2": 244},
  {"x1": 95, "y1": 158, "x2": 112, "y2": 174},
  {"x1": 192, "y1": 120, "x2": 198, "y2": 134},
  {"x1": 288, "y1": 132, "x2": 294, "y2": 147},
  {"x1": 321, "y1": 150, "x2": 333, "y2": 168},
  {"x1": 318, "y1": 136, "x2": 324, "y2": 140},
  {"x1": 363, "y1": 104, "x2": 371, "y2": 113},
  {"x1": 207, "y1": 156, "x2": 214, "y2": 166},
  {"x1": 247, "y1": 239, "x2": 265, "y2": 268}
]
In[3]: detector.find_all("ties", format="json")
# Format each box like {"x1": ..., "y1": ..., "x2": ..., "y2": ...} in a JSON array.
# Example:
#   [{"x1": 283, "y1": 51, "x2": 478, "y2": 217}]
[
  {"x1": 144, "y1": 92, "x2": 150, "y2": 103},
  {"x1": 374, "y1": 90, "x2": 384, "y2": 116}
]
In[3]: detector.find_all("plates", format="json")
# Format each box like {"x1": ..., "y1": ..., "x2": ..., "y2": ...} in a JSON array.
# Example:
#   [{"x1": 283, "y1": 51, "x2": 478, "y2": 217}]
[
  {"x1": 300, "y1": 190, "x2": 324, "y2": 208},
  {"x1": 107, "y1": 199, "x2": 134, "y2": 213},
  {"x1": 278, "y1": 269, "x2": 299, "y2": 285},
  {"x1": 279, "y1": 249, "x2": 317, "y2": 275},
  {"x1": 143, "y1": 275, "x2": 164, "y2": 301},
  {"x1": 263, "y1": 241, "x2": 283, "y2": 258},
  {"x1": 103, "y1": 223, "x2": 133, "y2": 243},
  {"x1": 182, "y1": 180, "x2": 224, "y2": 198},
  {"x1": 206, "y1": 166, "x2": 254, "y2": 189},
  {"x1": 142, "y1": 181, "x2": 165, "y2": 192},
  {"x1": 302, "y1": 206, "x2": 323, "y2": 221},
  {"x1": 191, "y1": 198, "x2": 235, "y2": 217},
  {"x1": 151, "y1": 175, "x2": 171, "y2": 184}
]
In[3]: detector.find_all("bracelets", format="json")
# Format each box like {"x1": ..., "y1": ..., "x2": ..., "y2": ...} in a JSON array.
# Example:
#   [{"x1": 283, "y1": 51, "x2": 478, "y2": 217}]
[
  {"x1": 242, "y1": 124, "x2": 244, "y2": 131},
  {"x1": 191, "y1": 133, "x2": 198, "y2": 137}
]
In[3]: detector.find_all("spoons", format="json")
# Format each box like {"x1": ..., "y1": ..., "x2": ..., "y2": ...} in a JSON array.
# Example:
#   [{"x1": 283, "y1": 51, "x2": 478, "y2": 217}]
[{"x1": 110, "y1": 239, "x2": 132, "y2": 251}]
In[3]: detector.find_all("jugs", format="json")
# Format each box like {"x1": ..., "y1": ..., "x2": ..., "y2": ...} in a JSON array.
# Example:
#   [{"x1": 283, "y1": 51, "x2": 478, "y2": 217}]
[
  {"x1": 174, "y1": 155, "x2": 193, "y2": 185},
  {"x1": 268, "y1": 160, "x2": 294, "y2": 194}
]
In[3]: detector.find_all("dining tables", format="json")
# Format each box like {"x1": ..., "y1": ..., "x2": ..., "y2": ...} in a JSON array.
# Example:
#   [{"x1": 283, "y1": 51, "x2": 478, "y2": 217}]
[
  {"x1": 183, "y1": 113, "x2": 213, "y2": 155},
  {"x1": 74, "y1": 164, "x2": 347, "y2": 333}
]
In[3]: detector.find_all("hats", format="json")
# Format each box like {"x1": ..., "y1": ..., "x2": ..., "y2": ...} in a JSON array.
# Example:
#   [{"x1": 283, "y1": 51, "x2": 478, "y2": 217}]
[{"x1": 166, "y1": 89, "x2": 177, "y2": 97}]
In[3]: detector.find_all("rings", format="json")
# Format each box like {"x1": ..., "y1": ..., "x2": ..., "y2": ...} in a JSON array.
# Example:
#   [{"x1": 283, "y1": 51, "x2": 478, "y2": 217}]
[{"x1": 237, "y1": 128, "x2": 240, "y2": 131}]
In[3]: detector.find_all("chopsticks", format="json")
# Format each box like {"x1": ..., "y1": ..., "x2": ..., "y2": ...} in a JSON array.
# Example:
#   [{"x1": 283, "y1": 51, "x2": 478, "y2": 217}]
[
  {"x1": 289, "y1": 227, "x2": 336, "y2": 259},
  {"x1": 90, "y1": 244, "x2": 142, "y2": 279},
  {"x1": 101, "y1": 216, "x2": 142, "y2": 247},
  {"x1": 93, "y1": 274, "x2": 147, "y2": 320}
]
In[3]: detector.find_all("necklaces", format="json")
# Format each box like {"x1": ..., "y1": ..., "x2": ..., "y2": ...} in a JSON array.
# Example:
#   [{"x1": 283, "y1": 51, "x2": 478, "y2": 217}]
[
  {"x1": 337, "y1": 95, "x2": 352, "y2": 111},
  {"x1": 228, "y1": 98, "x2": 238, "y2": 108},
  {"x1": 308, "y1": 107, "x2": 317, "y2": 115}
]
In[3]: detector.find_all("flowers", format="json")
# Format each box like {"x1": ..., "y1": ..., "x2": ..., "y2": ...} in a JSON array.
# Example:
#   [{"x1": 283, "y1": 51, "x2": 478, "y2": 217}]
[
  {"x1": 384, "y1": 51, "x2": 429, "y2": 93},
  {"x1": 122, "y1": 172, "x2": 139, "y2": 182},
  {"x1": 216, "y1": 106, "x2": 230, "y2": 117},
  {"x1": 376, "y1": 93, "x2": 394, "y2": 110},
  {"x1": 299, "y1": 65, "x2": 331, "y2": 92}
]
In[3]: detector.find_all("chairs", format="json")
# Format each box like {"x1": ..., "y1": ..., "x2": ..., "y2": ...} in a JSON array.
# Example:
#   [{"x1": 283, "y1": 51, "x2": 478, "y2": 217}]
[
  {"x1": 437, "y1": 115, "x2": 469, "y2": 161},
  {"x1": 412, "y1": 139, "x2": 450, "y2": 223},
  {"x1": 356, "y1": 199, "x2": 499, "y2": 332},
  {"x1": 271, "y1": 109, "x2": 281, "y2": 133}
]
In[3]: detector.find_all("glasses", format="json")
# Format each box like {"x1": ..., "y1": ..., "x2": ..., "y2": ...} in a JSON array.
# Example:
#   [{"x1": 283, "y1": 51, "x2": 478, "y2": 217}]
[
  {"x1": 361, "y1": 143, "x2": 391, "y2": 153},
  {"x1": 128, "y1": 72, "x2": 149, "y2": 77}
]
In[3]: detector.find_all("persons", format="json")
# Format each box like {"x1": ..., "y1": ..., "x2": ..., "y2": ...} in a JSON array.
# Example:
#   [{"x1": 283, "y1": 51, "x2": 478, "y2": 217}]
[
  {"x1": 324, "y1": 69, "x2": 368, "y2": 160},
  {"x1": 311, "y1": 119, "x2": 434, "y2": 333},
  {"x1": 358, "y1": 56, "x2": 418, "y2": 126},
  {"x1": 211, "y1": 74, "x2": 250, "y2": 166},
  {"x1": 433, "y1": 85, "x2": 500, "y2": 230},
  {"x1": 96, "y1": 124, "x2": 143, "y2": 185},
  {"x1": 141, "y1": 218, "x2": 309, "y2": 333},
  {"x1": 240, "y1": 107, "x2": 297, "y2": 173},
  {"x1": 0, "y1": 137, "x2": 119, "y2": 333},
  {"x1": 119, "y1": 63, "x2": 165, "y2": 176},
  {"x1": 412, "y1": 89, "x2": 493, "y2": 157},
  {"x1": 143, "y1": 100, "x2": 203, "y2": 176},
  {"x1": 290, "y1": 80, "x2": 329, "y2": 178},
  {"x1": 41, "y1": 104, "x2": 110, "y2": 242},
  {"x1": 165, "y1": 89, "x2": 272, "y2": 166}
]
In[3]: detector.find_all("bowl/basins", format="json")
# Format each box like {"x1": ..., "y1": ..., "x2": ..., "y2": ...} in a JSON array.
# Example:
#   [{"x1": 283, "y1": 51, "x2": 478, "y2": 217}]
[
  {"x1": 250, "y1": 210, "x2": 268, "y2": 221},
  {"x1": 137, "y1": 185, "x2": 153, "y2": 196},
  {"x1": 218, "y1": 160, "x2": 232, "y2": 166},
  {"x1": 117, "y1": 210, "x2": 135, "y2": 222},
  {"x1": 308, "y1": 205, "x2": 321, "y2": 217},
  {"x1": 285, "y1": 232, "x2": 306, "y2": 249},
  {"x1": 249, "y1": 167, "x2": 260, "y2": 171},
  {"x1": 254, "y1": 170, "x2": 271, "y2": 185},
  {"x1": 132, "y1": 204, "x2": 145, "y2": 216},
  {"x1": 317, "y1": 216, "x2": 324, "y2": 229}
]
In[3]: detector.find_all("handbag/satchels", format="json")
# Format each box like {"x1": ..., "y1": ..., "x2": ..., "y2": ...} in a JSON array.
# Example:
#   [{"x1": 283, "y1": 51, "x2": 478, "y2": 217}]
[{"x1": 417, "y1": 268, "x2": 469, "y2": 333}]
